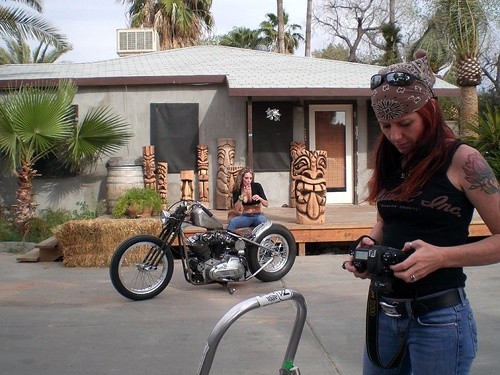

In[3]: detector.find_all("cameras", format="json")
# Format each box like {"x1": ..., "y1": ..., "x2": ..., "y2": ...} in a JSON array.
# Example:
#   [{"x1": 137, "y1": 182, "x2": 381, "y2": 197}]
[{"x1": 352, "y1": 244, "x2": 412, "y2": 291}]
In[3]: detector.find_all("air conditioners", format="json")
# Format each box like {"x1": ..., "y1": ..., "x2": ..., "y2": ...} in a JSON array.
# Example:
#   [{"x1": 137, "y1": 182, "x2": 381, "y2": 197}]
[{"x1": 117, "y1": 28, "x2": 159, "y2": 56}]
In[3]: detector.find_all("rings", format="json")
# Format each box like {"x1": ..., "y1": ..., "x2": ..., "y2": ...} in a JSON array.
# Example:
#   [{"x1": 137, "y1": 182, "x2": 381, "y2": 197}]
[
  {"x1": 342, "y1": 261, "x2": 353, "y2": 269},
  {"x1": 409, "y1": 242, "x2": 413, "y2": 247},
  {"x1": 411, "y1": 275, "x2": 416, "y2": 281}
]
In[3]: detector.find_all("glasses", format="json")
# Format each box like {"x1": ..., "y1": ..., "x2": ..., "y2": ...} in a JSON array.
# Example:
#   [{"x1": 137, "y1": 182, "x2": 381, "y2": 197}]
[{"x1": 369, "y1": 72, "x2": 414, "y2": 90}]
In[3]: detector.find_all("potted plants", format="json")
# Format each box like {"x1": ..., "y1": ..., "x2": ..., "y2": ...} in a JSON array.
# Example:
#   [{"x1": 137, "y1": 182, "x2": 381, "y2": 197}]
[{"x1": 110, "y1": 187, "x2": 164, "y2": 218}]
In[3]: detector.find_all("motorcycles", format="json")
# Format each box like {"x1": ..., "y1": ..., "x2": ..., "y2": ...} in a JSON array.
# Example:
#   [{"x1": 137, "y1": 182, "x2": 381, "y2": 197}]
[{"x1": 108, "y1": 198, "x2": 298, "y2": 301}]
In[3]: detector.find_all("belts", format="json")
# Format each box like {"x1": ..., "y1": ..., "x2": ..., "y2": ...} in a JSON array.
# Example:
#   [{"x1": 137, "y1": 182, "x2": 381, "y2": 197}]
[{"x1": 376, "y1": 288, "x2": 466, "y2": 319}]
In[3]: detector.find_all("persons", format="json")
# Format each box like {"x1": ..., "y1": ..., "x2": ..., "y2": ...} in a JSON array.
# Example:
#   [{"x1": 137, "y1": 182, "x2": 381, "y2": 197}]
[
  {"x1": 342, "y1": 49, "x2": 500, "y2": 375},
  {"x1": 227, "y1": 167, "x2": 268, "y2": 233}
]
